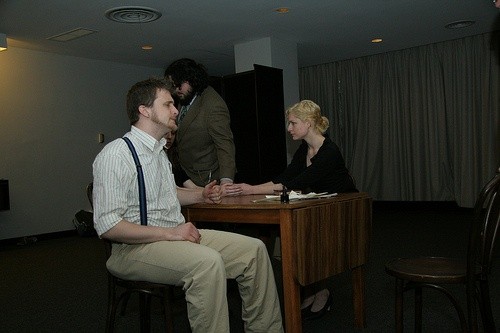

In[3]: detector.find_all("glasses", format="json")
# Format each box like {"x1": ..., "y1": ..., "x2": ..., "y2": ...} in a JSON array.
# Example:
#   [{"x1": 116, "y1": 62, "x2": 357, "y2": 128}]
[{"x1": 169, "y1": 79, "x2": 186, "y2": 89}]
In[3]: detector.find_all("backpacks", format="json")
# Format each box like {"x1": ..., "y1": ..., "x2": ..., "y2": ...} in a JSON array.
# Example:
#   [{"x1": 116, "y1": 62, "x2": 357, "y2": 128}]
[{"x1": 72, "y1": 210, "x2": 98, "y2": 236}]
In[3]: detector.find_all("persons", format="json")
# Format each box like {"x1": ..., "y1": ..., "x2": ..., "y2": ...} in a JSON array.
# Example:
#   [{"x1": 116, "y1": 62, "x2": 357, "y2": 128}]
[
  {"x1": 160, "y1": 121, "x2": 202, "y2": 189},
  {"x1": 163, "y1": 59, "x2": 238, "y2": 187},
  {"x1": 93, "y1": 79, "x2": 285, "y2": 333},
  {"x1": 223, "y1": 101, "x2": 359, "y2": 321}
]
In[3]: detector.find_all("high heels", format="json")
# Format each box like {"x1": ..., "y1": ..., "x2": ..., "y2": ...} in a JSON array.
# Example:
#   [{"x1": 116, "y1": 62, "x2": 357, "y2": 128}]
[{"x1": 301, "y1": 290, "x2": 337, "y2": 320}]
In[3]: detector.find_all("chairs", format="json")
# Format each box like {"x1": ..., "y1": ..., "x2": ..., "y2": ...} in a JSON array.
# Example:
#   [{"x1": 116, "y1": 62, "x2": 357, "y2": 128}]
[
  {"x1": 87, "y1": 182, "x2": 186, "y2": 333},
  {"x1": 387, "y1": 167, "x2": 500, "y2": 333}
]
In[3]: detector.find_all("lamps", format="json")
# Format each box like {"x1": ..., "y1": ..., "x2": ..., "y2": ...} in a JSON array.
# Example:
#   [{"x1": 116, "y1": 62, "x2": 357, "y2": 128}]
[{"x1": 0, "y1": 33, "x2": 7, "y2": 51}]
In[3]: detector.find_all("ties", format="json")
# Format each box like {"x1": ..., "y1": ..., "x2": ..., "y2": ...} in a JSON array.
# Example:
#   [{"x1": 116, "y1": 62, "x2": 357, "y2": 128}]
[{"x1": 179, "y1": 104, "x2": 187, "y2": 127}]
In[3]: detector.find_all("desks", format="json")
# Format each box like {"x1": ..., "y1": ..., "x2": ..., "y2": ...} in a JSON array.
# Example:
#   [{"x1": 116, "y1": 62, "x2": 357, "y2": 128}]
[{"x1": 181, "y1": 194, "x2": 373, "y2": 333}]
